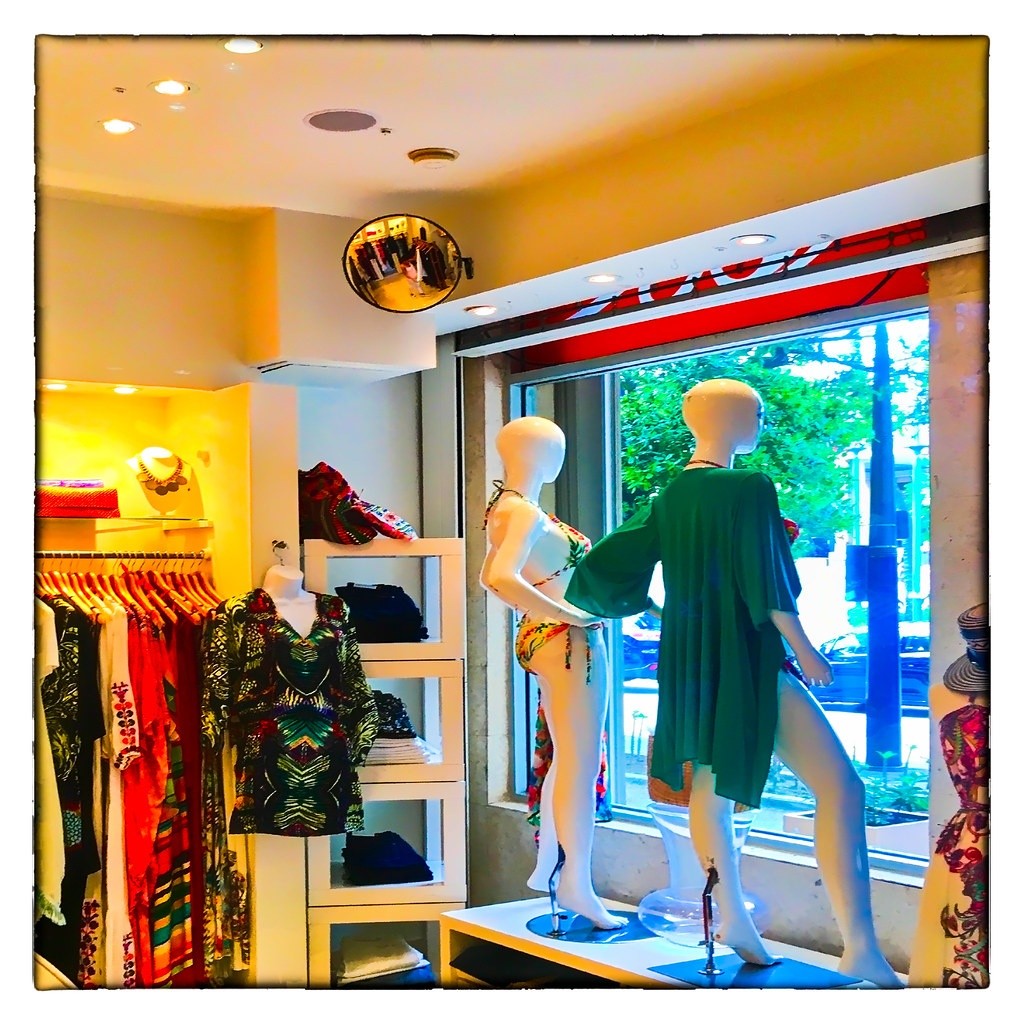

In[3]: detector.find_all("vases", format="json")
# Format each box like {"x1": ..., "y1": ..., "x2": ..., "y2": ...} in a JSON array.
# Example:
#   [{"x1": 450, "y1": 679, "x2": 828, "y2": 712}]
[{"x1": 637, "y1": 802, "x2": 771, "y2": 947}]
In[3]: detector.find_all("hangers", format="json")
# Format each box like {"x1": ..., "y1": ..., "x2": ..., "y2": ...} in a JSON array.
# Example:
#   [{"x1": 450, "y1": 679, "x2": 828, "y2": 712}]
[{"x1": 34, "y1": 549, "x2": 225, "y2": 626}]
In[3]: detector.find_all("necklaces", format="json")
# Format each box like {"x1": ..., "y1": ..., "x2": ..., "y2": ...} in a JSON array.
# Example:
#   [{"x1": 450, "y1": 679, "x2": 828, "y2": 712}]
[{"x1": 136, "y1": 452, "x2": 187, "y2": 495}]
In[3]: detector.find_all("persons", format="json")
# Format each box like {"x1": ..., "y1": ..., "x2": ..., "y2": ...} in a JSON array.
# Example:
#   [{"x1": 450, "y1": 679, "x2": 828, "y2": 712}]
[
  {"x1": 908, "y1": 597, "x2": 988, "y2": 989},
  {"x1": 200, "y1": 558, "x2": 380, "y2": 838},
  {"x1": 563, "y1": 376, "x2": 908, "y2": 990},
  {"x1": 478, "y1": 416, "x2": 626, "y2": 941},
  {"x1": 125, "y1": 447, "x2": 205, "y2": 519}
]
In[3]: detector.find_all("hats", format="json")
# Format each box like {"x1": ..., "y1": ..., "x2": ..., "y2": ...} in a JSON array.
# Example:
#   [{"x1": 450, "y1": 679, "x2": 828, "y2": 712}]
[{"x1": 943, "y1": 602, "x2": 991, "y2": 692}]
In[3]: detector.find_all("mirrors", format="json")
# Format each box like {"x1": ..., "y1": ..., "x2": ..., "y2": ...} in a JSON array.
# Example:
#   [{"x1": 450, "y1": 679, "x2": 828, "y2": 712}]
[{"x1": 340, "y1": 213, "x2": 462, "y2": 313}]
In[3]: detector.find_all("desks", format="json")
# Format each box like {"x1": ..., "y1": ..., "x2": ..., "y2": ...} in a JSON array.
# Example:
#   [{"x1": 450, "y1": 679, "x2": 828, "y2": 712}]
[{"x1": 441, "y1": 893, "x2": 909, "y2": 989}]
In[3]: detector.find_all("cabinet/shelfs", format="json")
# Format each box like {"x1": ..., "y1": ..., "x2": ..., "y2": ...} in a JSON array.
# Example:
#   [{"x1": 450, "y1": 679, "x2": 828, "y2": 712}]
[{"x1": 303, "y1": 536, "x2": 467, "y2": 988}]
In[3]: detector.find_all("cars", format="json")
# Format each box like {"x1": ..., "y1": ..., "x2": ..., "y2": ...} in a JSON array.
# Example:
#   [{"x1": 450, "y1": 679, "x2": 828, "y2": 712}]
[
  {"x1": 788, "y1": 631, "x2": 929, "y2": 708},
  {"x1": 622, "y1": 609, "x2": 661, "y2": 683}
]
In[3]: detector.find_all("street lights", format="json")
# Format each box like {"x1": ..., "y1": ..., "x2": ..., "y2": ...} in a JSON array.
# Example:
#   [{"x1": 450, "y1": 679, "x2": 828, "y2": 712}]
[
  {"x1": 906, "y1": 441, "x2": 928, "y2": 620},
  {"x1": 845, "y1": 439, "x2": 870, "y2": 544}
]
